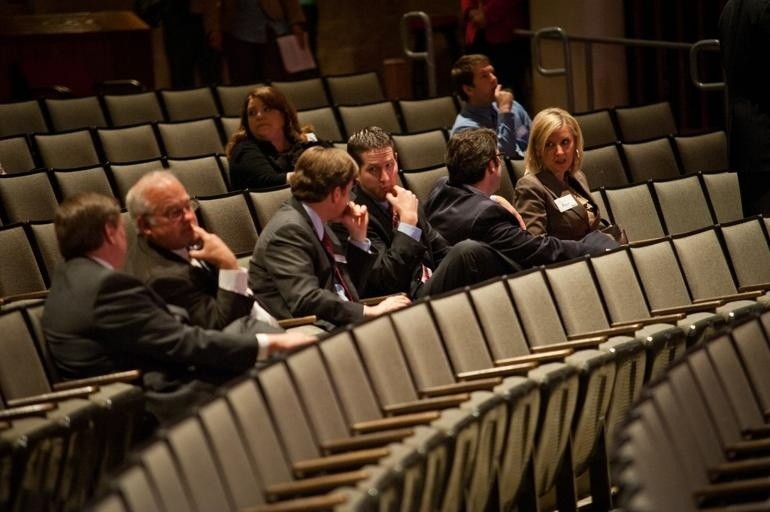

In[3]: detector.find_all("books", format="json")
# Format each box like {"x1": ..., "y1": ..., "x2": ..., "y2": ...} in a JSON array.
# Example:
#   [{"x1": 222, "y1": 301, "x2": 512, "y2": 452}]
[{"x1": 273, "y1": 30, "x2": 318, "y2": 74}]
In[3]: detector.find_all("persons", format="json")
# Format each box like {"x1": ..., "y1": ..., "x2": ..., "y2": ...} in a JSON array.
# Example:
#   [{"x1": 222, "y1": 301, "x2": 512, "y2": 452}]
[
  {"x1": 510, "y1": 106, "x2": 623, "y2": 243},
  {"x1": 448, "y1": 51, "x2": 536, "y2": 162},
  {"x1": 456, "y1": 0, "x2": 523, "y2": 110},
  {"x1": 247, "y1": 142, "x2": 413, "y2": 323},
  {"x1": 423, "y1": 125, "x2": 622, "y2": 274},
  {"x1": 217, "y1": 0, "x2": 318, "y2": 88},
  {"x1": 223, "y1": 84, "x2": 319, "y2": 192},
  {"x1": 39, "y1": 191, "x2": 329, "y2": 392},
  {"x1": 122, "y1": 170, "x2": 284, "y2": 332},
  {"x1": 344, "y1": 124, "x2": 523, "y2": 300}
]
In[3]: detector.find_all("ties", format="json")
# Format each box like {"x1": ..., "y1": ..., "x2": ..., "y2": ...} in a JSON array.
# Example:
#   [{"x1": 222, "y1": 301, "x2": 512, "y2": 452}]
[
  {"x1": 388, "y1": 201, "x2": 433, "y2": 283},
  {"x1": 187, "y1": 244, "x2": 212, "y2": 270},
  {"x1": 322, "y1": 225, "x2": 354, "y2": 302}
]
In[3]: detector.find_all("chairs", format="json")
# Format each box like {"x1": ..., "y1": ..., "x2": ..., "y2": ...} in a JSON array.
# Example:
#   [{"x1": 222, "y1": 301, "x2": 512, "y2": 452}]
[{"x1": 0, "y1": 70, "x2": 770, "y2": 511}]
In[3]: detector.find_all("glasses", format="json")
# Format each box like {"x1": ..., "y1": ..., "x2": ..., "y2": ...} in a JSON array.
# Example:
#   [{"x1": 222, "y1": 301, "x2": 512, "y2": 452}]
[
  {"x1": 144, "y1": 200, "x2": 199, "y2": 218},
  {"x1": 497, "y1": 152, "x2": 506, "y2": 160}
]
